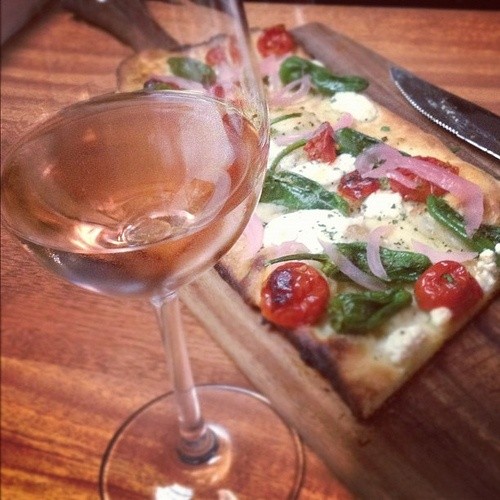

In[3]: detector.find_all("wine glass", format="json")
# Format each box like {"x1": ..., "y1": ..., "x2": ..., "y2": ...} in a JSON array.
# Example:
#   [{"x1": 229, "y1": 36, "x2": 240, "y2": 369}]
[{"x1": 0, "y1": 0, "x2": 304, "y2": 499}]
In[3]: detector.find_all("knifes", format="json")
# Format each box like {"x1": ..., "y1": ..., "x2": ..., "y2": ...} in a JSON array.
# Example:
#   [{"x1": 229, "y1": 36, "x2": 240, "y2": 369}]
[{"x1": 389, "y1": 66, "x2": 500, "y2": 160}]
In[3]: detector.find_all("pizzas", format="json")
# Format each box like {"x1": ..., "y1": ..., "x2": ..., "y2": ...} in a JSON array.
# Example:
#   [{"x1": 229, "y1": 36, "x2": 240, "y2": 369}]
[{"x1": 108, "y1": 23, "x2": 500, "y2": 422}]
